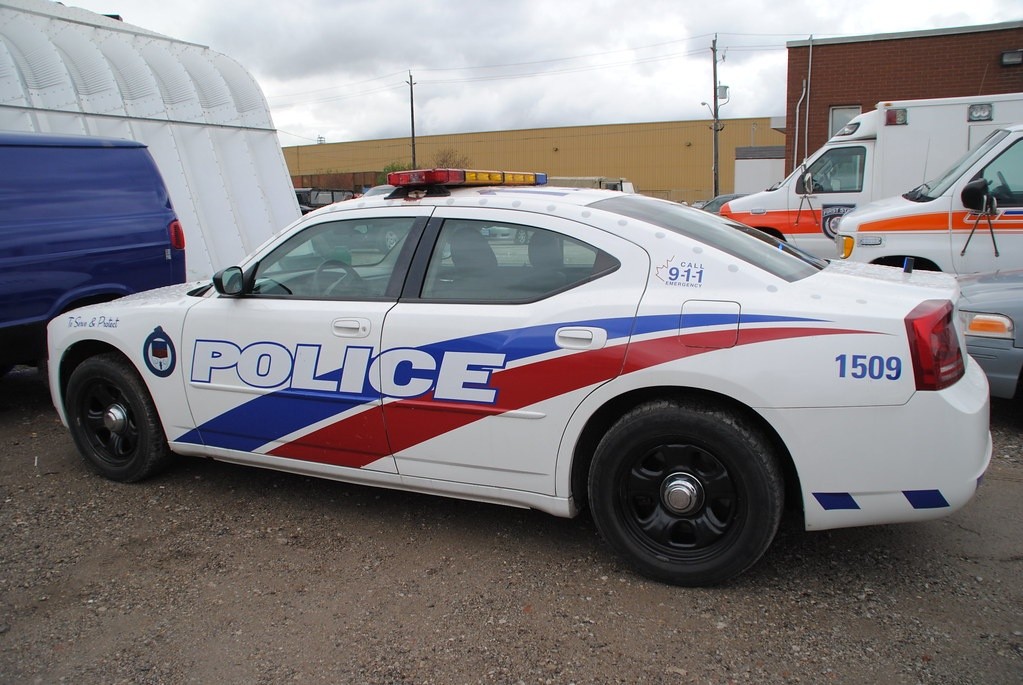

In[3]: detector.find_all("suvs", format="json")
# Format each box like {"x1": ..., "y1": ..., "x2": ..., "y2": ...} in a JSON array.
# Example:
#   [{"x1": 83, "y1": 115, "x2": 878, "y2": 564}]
[{"x1": 295, "y1": 187, "x2": 355, "y2": 216}]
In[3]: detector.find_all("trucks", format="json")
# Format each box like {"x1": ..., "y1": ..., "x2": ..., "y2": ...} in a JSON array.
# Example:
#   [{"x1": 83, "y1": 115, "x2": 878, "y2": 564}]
[
  {"x1": 836, "y1": 124, "x2": 1022, "y2": 279},
  {"x1": 719, "y1": 89, "x2": 1023, "y2": 260},
  {"x1": 538, "y1": 177, "x2": 635, "y2": 194}
]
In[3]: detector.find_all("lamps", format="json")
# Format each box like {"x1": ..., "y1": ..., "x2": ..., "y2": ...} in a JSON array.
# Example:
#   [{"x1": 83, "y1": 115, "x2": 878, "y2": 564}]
[{"x1": 1000, "y1": 49, "x2": 1023, "y2": 67}]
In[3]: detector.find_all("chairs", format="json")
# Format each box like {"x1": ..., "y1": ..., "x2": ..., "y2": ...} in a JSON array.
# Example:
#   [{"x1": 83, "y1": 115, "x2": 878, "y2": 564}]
[
  {"x1": 514, "y1": 231, "x2": 568, "y2": 292},
  {"x1": 447, "y1": 226, "x2": 519, "y2": 302}
]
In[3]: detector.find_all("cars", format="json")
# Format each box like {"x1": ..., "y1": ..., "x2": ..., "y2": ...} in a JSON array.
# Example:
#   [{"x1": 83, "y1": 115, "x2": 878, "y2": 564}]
[
  {"x1": 43, "y1": 168, "x2": 994, "y2": 585},
  {"x1": 480, "y1": 222, "x2": 534, "y2": 246},
  {"x1": 701, "y1": 193, "x2": 751, "y2": 215},
  {"x1": 310, "y1": 185, "x2": 471, "y2": 251},
  {"x1": 952, "y1": 268, "x2": 1023, "y2": 416}
]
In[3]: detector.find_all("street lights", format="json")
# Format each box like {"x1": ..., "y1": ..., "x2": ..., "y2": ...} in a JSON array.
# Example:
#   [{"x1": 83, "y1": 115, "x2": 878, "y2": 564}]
[{"x1": 701, "y1": 102, "x2": 719, "y2": 198}]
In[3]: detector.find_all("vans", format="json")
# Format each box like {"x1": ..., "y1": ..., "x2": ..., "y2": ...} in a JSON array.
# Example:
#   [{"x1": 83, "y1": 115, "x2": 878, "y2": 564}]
[{"x1": 1, "y1": 132, "x2": 186, "y2": 400}]
